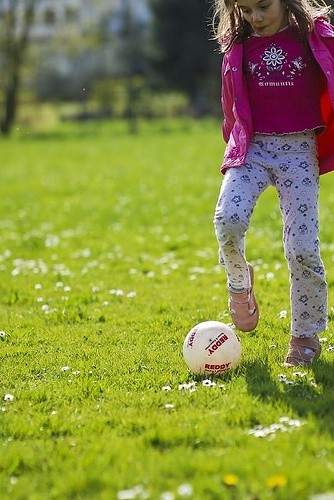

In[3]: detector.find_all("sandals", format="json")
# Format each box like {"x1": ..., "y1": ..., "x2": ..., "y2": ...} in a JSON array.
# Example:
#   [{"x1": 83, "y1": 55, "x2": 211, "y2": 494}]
[
  {"x1": 229, "y1": 262, "x2": 259, "y2": 332},
  {"x1": 284, "y1": 334, "x2": 322, "y2": 367}
]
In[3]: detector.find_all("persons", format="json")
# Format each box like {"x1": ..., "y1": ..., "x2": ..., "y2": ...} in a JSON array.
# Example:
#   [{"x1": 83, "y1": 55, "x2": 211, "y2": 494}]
[{"x1": 209, "y1": 0, "x2": 334, "y2": 372}]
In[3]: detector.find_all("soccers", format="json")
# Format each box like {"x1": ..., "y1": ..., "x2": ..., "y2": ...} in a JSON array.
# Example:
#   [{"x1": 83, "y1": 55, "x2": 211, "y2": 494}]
[{"x1": 183, "y1": 321, "x2": 241, "y2": 376}]
